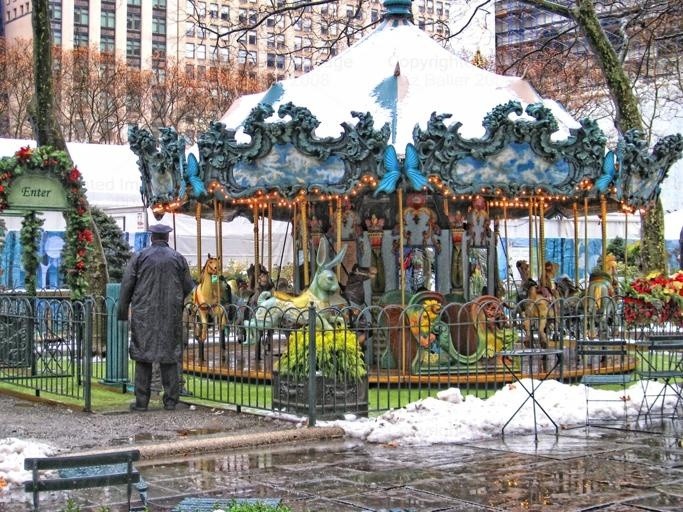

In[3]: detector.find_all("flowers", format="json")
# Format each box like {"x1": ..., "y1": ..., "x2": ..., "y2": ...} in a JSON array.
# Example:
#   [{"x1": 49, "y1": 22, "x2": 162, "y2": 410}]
[
  {"x1": 622, "y1": 270, "x2": 683, "y2": 328},
  {"x1": 0, "y1": 145, "x2": 100, "y2": 301}
]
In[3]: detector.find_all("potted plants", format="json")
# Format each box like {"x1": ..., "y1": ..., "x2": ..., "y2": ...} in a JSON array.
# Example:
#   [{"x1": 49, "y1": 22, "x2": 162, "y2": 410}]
[{"x1": 272, "y1": 324, "x2": 368, "y2": 422}]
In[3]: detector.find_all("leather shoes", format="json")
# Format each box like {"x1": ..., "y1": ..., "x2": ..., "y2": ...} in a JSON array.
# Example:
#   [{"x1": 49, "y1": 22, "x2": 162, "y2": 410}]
[
  {"x1": 129, "y1": 400, "x2": 147, "y2": 412},
  {"x1": 151, "y1": 385, "x2": 194, "y2": 410}
]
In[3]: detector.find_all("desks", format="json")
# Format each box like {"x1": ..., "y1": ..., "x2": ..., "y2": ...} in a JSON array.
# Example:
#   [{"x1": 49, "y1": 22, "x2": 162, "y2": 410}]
[
  {"x1": 494, "y1": 348, "x2": 563, "y2": 440},
  {"x1": 171, "y1": 497, "x2": 283, "y2": 512},
  {"x1": 634, "y1": 340, "x2": 683, "y2": 416}
]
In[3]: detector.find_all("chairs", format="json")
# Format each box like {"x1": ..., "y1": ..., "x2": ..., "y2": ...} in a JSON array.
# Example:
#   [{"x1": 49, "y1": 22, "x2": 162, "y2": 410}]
[
  {"x1": 577, "y1": 341, "x2": 630, "y2": 437},
  {"x1": 634, "y1": 336, "x2": 683, "y2": 425},
  {"x1": 24, "y1": 450, "x2": 149, "y2": 512}
]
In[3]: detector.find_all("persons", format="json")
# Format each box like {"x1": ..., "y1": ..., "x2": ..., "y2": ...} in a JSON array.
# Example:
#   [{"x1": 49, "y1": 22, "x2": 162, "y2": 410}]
[{"x1": 116, "y1": 223, "x2": 197, "y2": 414}]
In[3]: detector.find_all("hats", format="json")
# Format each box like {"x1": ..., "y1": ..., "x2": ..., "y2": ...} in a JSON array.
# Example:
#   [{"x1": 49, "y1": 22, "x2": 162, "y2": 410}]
[{"x1": 148, "y1": 224, "x2": 174, "y2": 235}]
